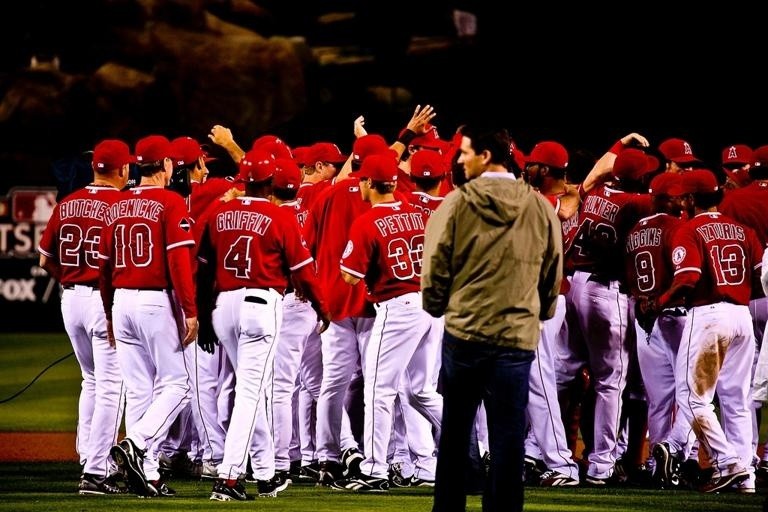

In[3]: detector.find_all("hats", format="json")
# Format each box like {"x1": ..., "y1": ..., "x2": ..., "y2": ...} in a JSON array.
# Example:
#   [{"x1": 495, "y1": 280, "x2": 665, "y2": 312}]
[
  {"x1": 236, "y1": 124, "x2": 465, "y2": 193},
  {"x1": 610, "y1": 139, "x2": 768, "y2": 198},
  {"x1": 134, "y1": 135, "x2": 184, "y2": 164},
  {"x1": 199, "y1": 149, "x2": 216, "y2": 164},
  {"x1": 506, "y1": 137, "x2": 528, "y2": 172},
  {"x1": 524, "y1": 142, "x2": 569, "y2": 168},
  {"x1": 93, "y1": 140, "x2": 135, "y2": 171},
  {"x1": 169, "y1": 137, "x2": 203, "y2": 168}
]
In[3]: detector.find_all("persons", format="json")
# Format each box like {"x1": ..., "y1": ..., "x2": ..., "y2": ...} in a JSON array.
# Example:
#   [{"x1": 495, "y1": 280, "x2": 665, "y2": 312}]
[
  {"x1": 39, "y1": 102, "x2": 768, "y2": 502},
  {"x1": 420, "y1": 117, "x2": 564, "y2": 510}
]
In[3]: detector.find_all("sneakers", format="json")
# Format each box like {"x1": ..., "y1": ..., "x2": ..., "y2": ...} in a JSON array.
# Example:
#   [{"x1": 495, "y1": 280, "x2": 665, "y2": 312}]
[
  {"x1": 78, "y1": 437, "x2": 435, "y2": 503},
  {"x1": 478, "y1": 453, "x2": 491, "y2": 490},
  {"x1": 523, "y1": 442, "x2": 768, "y2": 494}
]
